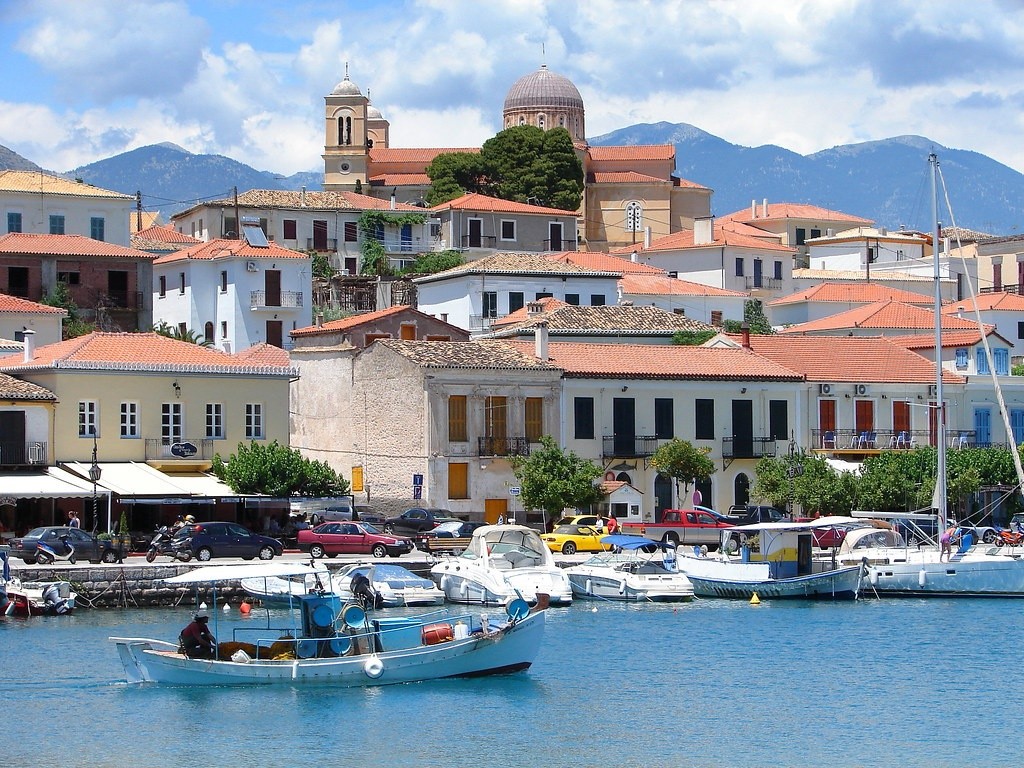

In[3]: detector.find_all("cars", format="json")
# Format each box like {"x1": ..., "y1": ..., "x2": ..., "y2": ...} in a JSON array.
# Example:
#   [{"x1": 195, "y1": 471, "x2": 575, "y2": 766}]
[
  {"x1": 310, "y1": 503, "x2": 384, "y2": 526},
  {"x1": 538, "y1": 524, "x2": 622, "y2": 556},
  {"x1": 382, "y1": 508, "x2": 466, "y2": 543},
  {"x1": 691, "y1": 503, "x2": 1024, "y2": 558},
  {"x1": 551, "y1": 514, "x2": 622, "y2": 536},
  {"x1": 172, "y1": 521, "x2": 284, "y2": 562},
  {"x1": 297, "y1": 520, "x2": 415, "y2": 559},
  {"x1": 6, "y1": 525, "x2": 120, "y2": 565},
  {"x1": 414, "y1": 520, "x2": 492, "y2": 556}
]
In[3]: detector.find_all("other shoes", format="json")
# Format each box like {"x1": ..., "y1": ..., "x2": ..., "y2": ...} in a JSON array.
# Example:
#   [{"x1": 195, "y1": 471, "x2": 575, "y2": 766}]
[{"x1": 170, "y1": 559, "x2": 175, "y2": 562}]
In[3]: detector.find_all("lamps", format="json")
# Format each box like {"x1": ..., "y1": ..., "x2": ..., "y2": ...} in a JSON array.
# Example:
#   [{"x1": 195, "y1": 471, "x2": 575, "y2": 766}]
[
  {"x1": 12, "y1": 402, "x2": 16, "y2": 406},
  {"x1": 985, "y1": 398, "x2": 1008, "y2": 415},
  {"x1": 845, "y1": 394, "x2": 851, "y2": 399},
  {"x1": 622, "y1": 385, "x2": 628, "y2": 392},
  {"x1": 882, "y1": 395, "x2": 887, "y2": 399},
  {"x1": 173, "y1": 379, "x2": 178, "y2": 387},
  {"x1": 918, "y1": 395, "x2": 924, "y2": 399},
  {"x1": 175, "y1": 385, "x2": 181, "y2": 398},
  {"x1": 741, "y1": 388, "x2": 747, "y2": 394}
]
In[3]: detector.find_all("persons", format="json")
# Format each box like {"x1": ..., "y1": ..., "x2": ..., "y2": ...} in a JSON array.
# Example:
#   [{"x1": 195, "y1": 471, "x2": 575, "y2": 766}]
[
  {"x1": 174, "y1": 514, "x2": 195, "y2": 528},
  {"x1": 594, "y1": 512, "x2": 603, "y2": 533},
  {"x1": 68, "y1": 511, "x2": 80, "y2": 529},
  {"x1": 939, "y1": 524, "x2": 961, "y2": 562},
  {"x1": 497, "y1": 512, "x2": 503, "y2": 525},
  {"x1": 607, "y1": 511, "x2": 618, "y2": 535},
  {"x1": 241, "y1": 511, "x2": 326, "y2": 533},
  {"x1": 181, "y1": 610, "x2": 218, "y2": 658},
  {"x1": 545, "y1": 519, "x2": 554, "y2": 533},
  {"x1": 0, "y1": 521, "x2": 7, "y2": 532}
]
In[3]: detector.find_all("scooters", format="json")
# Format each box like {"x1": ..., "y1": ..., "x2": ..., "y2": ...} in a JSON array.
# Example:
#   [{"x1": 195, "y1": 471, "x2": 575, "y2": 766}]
[{"x1": 34, "y1": 528, "x2": 78, "y2": 565}]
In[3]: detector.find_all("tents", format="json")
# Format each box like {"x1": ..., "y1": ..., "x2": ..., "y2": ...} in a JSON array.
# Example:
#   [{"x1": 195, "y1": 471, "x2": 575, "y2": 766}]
[
  {"x1": 0, "y1": 464, "x2": 113, "y2": 535},
  {"x1": 57, "y1": 461, "x2": 204, "y2": 534}
]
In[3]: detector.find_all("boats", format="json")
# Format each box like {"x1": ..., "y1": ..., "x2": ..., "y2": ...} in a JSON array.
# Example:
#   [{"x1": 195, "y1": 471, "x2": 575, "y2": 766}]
[
  {"x1": 312, "y1": 563, "x2": 447, "y2": 609},
  {"x1": 674, "y1": 518, "x2": 868, "y2": 602},
  {"x1": 106, "y1": 562, "x2": 548, "y2": 688},
  {"x1": 430, "y1": 521, "x2": 574, "y2": 612},
  {"x1": 0, "y1": 577, "x2": 78, "y2": 618},
  {"x1": 239, "y1": 576, "x2": 326, "y2": 608},
  {"x1": 561, "y1": 534, "x2": 696, "y2": 603}
]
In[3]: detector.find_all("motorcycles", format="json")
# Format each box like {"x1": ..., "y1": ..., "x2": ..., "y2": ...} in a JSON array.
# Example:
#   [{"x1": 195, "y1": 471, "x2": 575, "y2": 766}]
[{"x1": 145, "y1": 522, "x2": 193, "y2": 563}]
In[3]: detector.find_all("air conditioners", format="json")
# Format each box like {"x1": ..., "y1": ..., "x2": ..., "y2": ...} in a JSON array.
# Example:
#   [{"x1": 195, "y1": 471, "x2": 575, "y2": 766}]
[
  {"x1": 820, "y1": 384, "x2": 835, "y2": 395},
  {"x1": 855, "y1": 385, "x2": 870, "y2": 396}
]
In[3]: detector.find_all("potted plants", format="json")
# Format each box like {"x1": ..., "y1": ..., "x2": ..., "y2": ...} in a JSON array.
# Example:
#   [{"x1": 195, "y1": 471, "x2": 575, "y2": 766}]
[{"x1": 97, "y1": 532, "x2": 112, "y2": 547}]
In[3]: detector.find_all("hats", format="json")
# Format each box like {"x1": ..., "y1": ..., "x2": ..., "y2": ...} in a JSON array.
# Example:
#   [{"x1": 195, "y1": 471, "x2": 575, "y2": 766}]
[{"x1": 196, "y1": 610, "x2": 211, "y2": 618}]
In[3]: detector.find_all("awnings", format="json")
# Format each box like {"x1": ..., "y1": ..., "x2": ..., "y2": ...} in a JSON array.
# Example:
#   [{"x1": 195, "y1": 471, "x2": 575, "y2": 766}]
[{"x1": 161, "y1": 471, "x2": 274, "y2": 497}]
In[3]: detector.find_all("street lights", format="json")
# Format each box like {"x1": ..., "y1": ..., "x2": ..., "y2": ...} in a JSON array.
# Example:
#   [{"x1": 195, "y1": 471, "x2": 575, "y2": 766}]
[
  {"x1": 89, "y1": 428, "x2": 102, "y2": 564},
  {"x1": 786, "y1": 427, "x2": 804, "y2": 522}
]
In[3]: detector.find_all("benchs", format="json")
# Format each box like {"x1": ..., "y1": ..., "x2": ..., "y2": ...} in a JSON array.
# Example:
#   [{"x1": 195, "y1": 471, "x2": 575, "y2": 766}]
[{"x1": 427, "y1": 537, "x2": 471, "y2": 558}]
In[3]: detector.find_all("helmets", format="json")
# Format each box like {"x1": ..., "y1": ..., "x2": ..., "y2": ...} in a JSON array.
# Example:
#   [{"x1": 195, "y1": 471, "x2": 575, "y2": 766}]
[
  {"x1": 175, "y1": 515, "x2": 183, "y2": 521},
  {"x1": 186, "y1": 515, "x2": 195, "y2": 523}
]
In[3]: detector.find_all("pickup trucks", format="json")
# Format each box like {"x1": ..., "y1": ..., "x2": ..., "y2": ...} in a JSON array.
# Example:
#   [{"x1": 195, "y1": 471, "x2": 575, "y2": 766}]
[{"x1": 620, "y1": 507, "x2": 743, "y2": 555}]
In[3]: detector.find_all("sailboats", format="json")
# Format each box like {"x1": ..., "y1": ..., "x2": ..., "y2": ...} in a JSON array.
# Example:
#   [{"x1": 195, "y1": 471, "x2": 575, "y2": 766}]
[{"x1": 836, "y1": 150, "x2": 1024, "y2": 599}]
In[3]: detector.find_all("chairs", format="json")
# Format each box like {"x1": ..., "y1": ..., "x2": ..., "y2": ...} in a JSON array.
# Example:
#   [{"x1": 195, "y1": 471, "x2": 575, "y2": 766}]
[
  {"x1": 952, "y1": 432, "x2": 970, "y2": 450},
  {"x1": 889, "y1": 431, "x2": 919, "y2": 449},
  {"x1": 859, "y1": 431, "x2": 878, "y2": 448},
  {"x1": 822, "y1": 431, "x2": 837, "y2": 450},
  {"x1": 850, "y1": 431, "x2": 869, "y2": 449},
  {"x1": 340, "y1": 528, "x2": 348, "y2": 534}
]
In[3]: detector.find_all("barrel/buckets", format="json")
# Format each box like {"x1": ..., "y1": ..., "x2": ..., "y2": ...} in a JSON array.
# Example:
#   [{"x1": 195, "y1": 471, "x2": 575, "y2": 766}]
[{"x1": 420, "y1": 622, "x2": 454, "y2": 645}]
